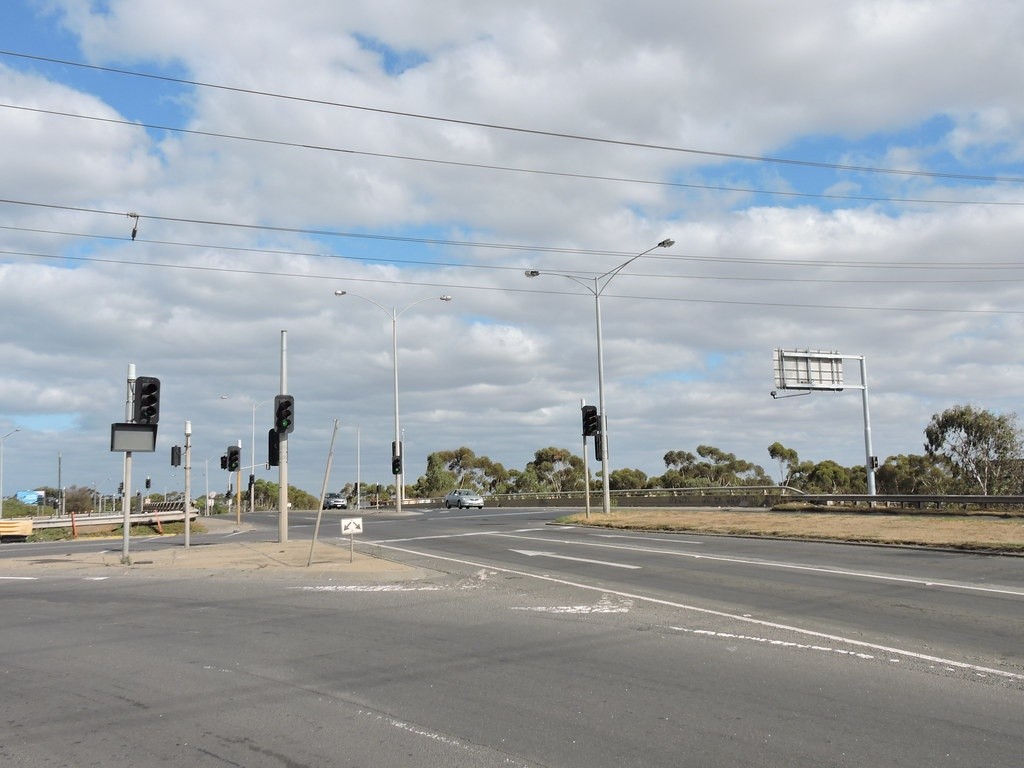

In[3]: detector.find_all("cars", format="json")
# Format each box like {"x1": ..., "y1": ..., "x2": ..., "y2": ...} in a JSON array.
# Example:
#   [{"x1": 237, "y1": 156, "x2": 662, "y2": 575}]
[
  {"x1": 444, "y1": 489, "x2": 484, "y2": 509},
  {"x1": 323, "y1": 493, "x2": 348, "y2": 511}
]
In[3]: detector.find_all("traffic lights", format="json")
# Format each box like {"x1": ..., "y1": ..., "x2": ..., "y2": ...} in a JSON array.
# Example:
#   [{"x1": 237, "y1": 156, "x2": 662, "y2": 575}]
[
  {"x1": 135, "y1": 376, "x2": 162, "y2": 425},
  {"x1": 376, "y1": 485, "x2": 380, "y2": 493},
  {"x1": 249, "y1": 475, "x2": 255, "y2": 484},
  {"x1": 582, "y1": 405, "x2": 601, "y2": 437},
  {"x1": 392, "y1": 456, "x2": 402, "y2": 474},
  {"x1": 226, "y1": 445, "x2": 241, "y2": 472},
  {"x1": 120, "y1": 482, "x2": 124, "y2": 490},
  {"x1": 226, "y1": 493, "x2": 228, "y2": 498},
  {"x1": 273, "y1": 395, "x2": 296, "y2": 434},
  {"x1": 146, "y1": 479, "x2": 151, "y2": 488},
  {"x1": 118, "y1": 496, "x2": 121, "y2": 501},
  {"x1": 227, "y1": 491, "x2": 231, "y2": 499},
  {"x1": 110, "y1": 421, "x2": 159, "y2": 453},
  {"x1": 118, "y1": 487, "x2": 122, "y2": 494}
]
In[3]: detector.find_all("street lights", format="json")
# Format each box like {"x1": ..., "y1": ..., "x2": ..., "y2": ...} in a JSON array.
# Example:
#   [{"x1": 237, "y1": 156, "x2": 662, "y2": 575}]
[
  {"x1": 0, "y1": 428, "x2": 23, "y2": 519},
  {"x1": 221, "y1": 395, "x2": 274, "y2": 511},
  {"x1": 523, "y1": 238, "x2": 676, "y2": 517},
  {"x1": 331, "y1": 287, "x2": 454, "y2": 513},
  {"x1": 332, "y1": 418, "x2": 363, "y2": 510}
]
